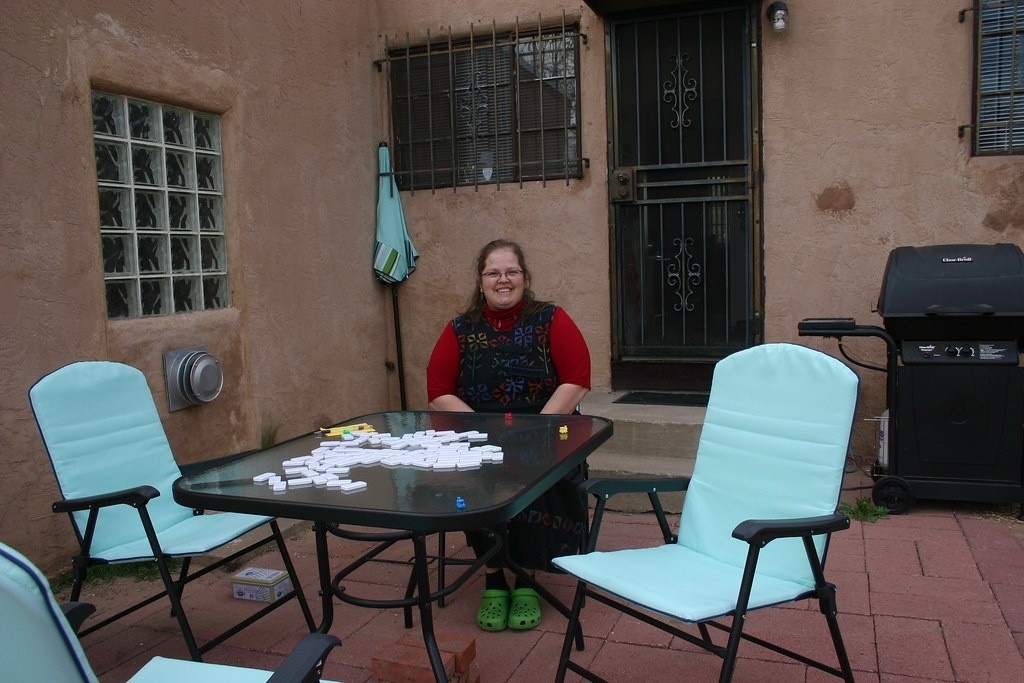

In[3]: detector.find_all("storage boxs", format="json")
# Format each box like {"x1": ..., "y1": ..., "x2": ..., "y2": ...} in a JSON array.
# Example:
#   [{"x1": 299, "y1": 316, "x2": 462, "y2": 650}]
[{"x1": 229, "y1": 567, "x2": 290, "y2": 604}]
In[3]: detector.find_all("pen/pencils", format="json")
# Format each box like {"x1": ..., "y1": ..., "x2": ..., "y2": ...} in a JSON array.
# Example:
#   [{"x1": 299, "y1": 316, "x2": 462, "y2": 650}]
[{"x1": 314, "y1": 424, "x2": 375, "y2": 435}]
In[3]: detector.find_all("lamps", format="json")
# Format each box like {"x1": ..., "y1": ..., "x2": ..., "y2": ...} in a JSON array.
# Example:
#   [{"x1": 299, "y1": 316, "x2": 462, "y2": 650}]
[{"x1": 767, "y1": 1, "x2": 789, "y2": 33}]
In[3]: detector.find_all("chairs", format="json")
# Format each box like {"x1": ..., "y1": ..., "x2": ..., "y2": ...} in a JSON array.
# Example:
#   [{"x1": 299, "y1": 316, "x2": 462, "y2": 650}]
[
  {"x1": 550, "y1": 342, "x2": 862, "y2": 683},
  {"x1": 28, "y1": 358, "x2": 317, "y2": 663},
  {"x1": 0, "y1": 541, "x2": 343, "y2": 683},
  {"x1": 438, "y1": 403, "x2": 580, "y2": 608}
]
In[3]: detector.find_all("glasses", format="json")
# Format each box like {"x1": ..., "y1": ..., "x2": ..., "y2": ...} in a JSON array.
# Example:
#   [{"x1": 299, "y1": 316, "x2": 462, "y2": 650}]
[{"x1": 481, "y1": 270, "x2": 524, "y2": 279}]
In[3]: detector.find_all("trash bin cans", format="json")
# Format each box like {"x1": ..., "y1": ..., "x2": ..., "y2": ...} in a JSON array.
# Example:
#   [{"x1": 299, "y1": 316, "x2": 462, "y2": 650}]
[{"x1": 876, "y1": 240, "x2": 1024, "y2": 518}]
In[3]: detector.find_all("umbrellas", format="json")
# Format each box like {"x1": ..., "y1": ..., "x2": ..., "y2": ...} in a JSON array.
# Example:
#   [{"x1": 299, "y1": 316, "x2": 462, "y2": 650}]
[{"x1": 374, "y1": 141, "x2": 420, "y2": 411}]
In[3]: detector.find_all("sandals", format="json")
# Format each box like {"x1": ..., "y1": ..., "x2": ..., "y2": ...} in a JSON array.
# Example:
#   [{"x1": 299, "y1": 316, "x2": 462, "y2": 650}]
[
  {"x1": 508, "y1": 588, "x2": 541, "y2": 629},
  {"x1": 477, "y1": 589, "x2": 511, "y2": 632}
]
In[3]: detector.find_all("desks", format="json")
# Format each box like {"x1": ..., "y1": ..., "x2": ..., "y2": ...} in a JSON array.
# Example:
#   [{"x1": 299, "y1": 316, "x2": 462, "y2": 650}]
[{"x1": 173, "y1": 411, "x2": 614, "y2": 683}]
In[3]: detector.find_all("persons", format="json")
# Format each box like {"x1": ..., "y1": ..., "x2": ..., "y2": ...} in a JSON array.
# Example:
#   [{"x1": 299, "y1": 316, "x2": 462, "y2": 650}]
[{"x1": 426, "y1": 239, "x2": 591, "y2": 631}]
